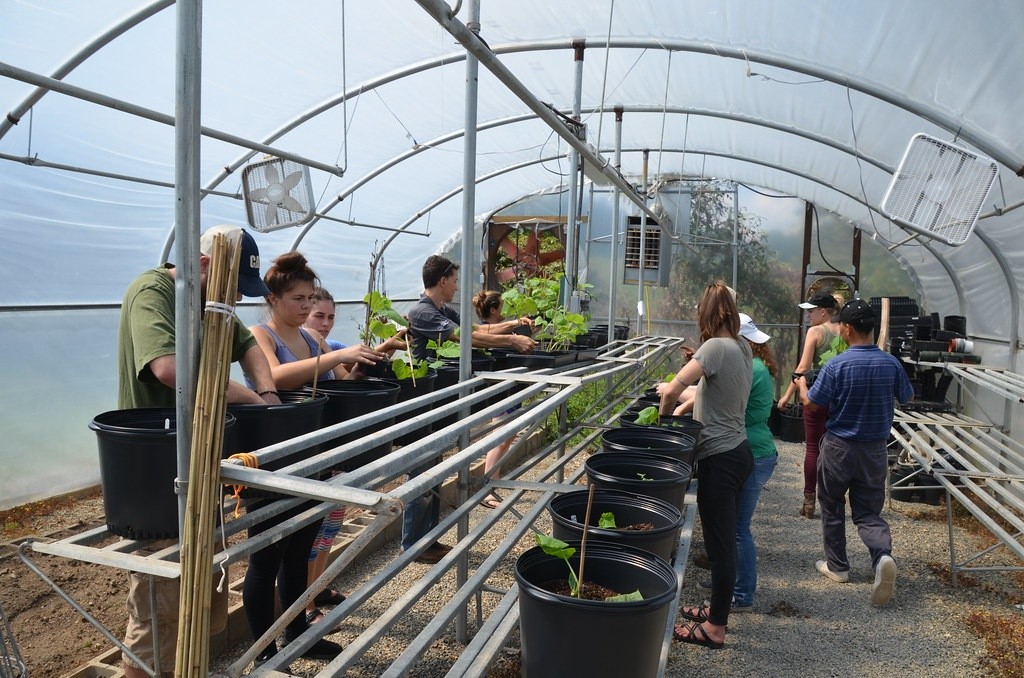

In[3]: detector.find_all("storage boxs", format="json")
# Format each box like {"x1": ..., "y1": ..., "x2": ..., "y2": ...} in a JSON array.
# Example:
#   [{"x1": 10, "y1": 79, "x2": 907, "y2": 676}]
[{"x1": 868, "y1": 296, "x2": 932, "y2": 397}]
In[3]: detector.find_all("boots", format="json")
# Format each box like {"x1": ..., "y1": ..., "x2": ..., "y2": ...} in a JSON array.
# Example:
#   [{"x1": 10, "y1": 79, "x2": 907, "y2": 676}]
[{"x1": 800, "y1": 491, "x2": 816, "y2": 518}]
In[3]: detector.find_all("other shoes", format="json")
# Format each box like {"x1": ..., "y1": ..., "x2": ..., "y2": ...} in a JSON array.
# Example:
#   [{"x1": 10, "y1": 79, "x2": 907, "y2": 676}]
[
  {"x1": 692, "y1": 555, "x2": 709, "y2": 570},
  {"x1": 281, "y1": 632, "x2": 344, "y2": 659},
  {"x1": 399, "y1": 540, "x2": 454, "y2": 565},
  {"x1": 253, "y1": 659, "x2": 294, "y2": 677}
]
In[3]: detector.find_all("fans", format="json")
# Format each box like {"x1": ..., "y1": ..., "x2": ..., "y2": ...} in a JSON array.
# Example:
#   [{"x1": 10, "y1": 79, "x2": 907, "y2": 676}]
[
  {"x1": 880, "y1": 134, "x2": 1001, "y2": 247},
  {"x1": 241, "y1": 160, "x2": 316, "y2": 232}
]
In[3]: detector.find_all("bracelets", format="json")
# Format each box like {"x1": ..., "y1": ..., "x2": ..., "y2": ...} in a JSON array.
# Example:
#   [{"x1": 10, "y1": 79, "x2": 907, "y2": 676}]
[{"x1": 259, "y1": 391, "x2": 281, "y2": 397}]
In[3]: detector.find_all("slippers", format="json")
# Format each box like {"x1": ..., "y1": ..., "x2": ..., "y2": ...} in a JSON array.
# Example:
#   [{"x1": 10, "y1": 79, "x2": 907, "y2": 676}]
[
  {"x1": 680, "y1": 604, "x2": 709, "y2": 622},
  {"x1": 673, "y1": 621, "x2": 724, "y2": 649},
  {"x1": 480, "y1": 497, "x2": 501, "y2": 509}
]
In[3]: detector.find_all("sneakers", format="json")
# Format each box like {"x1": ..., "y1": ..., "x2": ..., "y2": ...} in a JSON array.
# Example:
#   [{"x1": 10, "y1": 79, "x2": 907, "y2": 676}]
[
  {"x1": 696, "y1": 581, "x2": 713, "y2": 593},
  {"x1": 871, "y1": 555, "x2": 897, "y2": 603},
  {"x1": 704, "y1": 595, "x2": 752, "y2": 612},
  {"x1": 815, "y1": 560, "x2": 848, "y2": 582}
]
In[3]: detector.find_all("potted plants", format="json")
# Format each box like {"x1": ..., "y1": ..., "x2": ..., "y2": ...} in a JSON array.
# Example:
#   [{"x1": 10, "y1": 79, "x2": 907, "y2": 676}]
[{"x1": 89, "y1": 266, "x2": 705, "y2": 678}]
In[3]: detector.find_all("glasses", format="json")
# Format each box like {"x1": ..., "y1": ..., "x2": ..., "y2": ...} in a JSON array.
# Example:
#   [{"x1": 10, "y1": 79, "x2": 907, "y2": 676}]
[{"x1": 437, "y1": 261, "x2": 456, "y2": 282}]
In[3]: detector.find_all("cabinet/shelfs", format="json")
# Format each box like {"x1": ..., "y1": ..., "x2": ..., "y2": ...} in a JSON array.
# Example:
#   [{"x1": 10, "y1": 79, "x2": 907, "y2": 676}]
[
  {"x1": 889, "y1": 357, "x2": 1024, "y2": 589},
  {"x1": 15, "y1": 336, "x2": 685, "y2": 678}
]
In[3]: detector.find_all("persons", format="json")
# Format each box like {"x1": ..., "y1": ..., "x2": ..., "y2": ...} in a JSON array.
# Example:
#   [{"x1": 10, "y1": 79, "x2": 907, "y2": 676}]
[
  {"x1": 243, "y1": 252, "x2": 386, "y2": 673},
  {"x1": 399, "y1": 255, "x2": 539, "y2": 563},
  {"x1": 794, "y1": 298, "x2": 915, "y2": 604},
  {"x1": 777, "y1": 291, "x2": 850, "y2": 518},
  {"x1": 301, "y1": 287, "x2": 411, "y2": 633},
  {"x1": 471, "y1": 291, "x2": 541, "y2": 510},
  {"x1": 656, "y1": 313, "x2": 778, "y2": 612},
  {"x1": 659, "y1": 280, "x2": 754, "y2": 648},
  {"x1": 117, "y1": 224, "x2": 282, "y2": 678}
]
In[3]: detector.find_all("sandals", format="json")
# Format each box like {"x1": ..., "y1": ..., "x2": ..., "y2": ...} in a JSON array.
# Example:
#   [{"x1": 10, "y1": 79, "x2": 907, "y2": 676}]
[
  {"x1": 304, "y1": 610, "x2": 341, "y2": 633},
  {"x1": 313, "y1": 587, "x2": 346, "y2": 607}
]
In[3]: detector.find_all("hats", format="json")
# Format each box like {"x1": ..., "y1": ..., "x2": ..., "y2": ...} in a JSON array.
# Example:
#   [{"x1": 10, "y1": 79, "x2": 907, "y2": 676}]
[
  {"x1": 694, "y1": 286, "x2": 737, "y2": 312},
  {"x1": 830, "y1": 297, "x2": 876, "y2": 326},
  {"x1": 736, "y1": 312, "x2": 771, "y2": 344},
  {"x1": 797, "y1": 291, "x2": 838, "y2": 310},
  {"x1": 199, "y1": 224, "x2": 271, "y2": 296}
]
